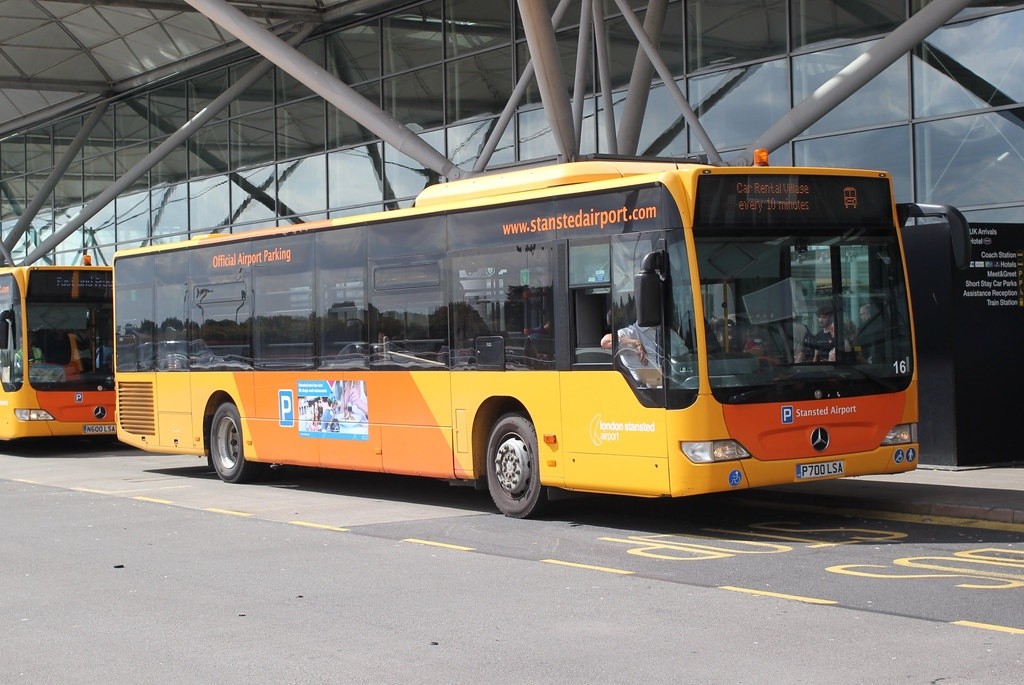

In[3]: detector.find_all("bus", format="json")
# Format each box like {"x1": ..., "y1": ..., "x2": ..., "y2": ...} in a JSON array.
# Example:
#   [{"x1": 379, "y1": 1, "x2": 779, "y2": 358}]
[
  {"x1": 111, "y1": 148, "x2": 919, "y2": 521},
  {"x1": 0, "y1": 255, "x2": 118, "y2": 442}
]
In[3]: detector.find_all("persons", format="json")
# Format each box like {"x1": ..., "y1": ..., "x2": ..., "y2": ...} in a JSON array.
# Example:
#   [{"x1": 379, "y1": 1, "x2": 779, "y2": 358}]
[
  {"x1": 600, "y1": 320, "x2": 690, "y2": 386},
  {"x1": 437, "y1": 301, "x2": 876, "y2": 365},
  {"x1": 11, "y1": 334, "x2": 113, "y2": 375}
]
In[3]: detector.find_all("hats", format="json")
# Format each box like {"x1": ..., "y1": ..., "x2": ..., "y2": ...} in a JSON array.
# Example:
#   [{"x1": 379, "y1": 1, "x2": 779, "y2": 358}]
[
  {"x1": 816, "y1": 306, "x2": 834, "y2": 315},
  {"x1": 20, "y1": 327, "x2": 34, "y2": 336}
]
